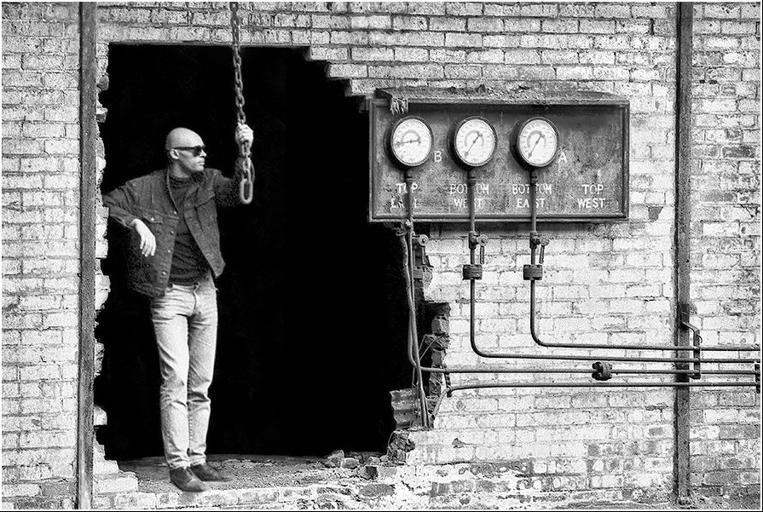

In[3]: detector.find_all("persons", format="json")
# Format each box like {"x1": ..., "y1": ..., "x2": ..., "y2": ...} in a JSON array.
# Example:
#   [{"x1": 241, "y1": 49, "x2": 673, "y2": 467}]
[{"x1": 103, "y1": 122, "x2": 258, "y2": 492}]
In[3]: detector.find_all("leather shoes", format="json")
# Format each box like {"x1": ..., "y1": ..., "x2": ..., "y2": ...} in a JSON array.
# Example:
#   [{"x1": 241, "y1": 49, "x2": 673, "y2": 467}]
[
  {"x1": 191, "y1": 463, "x2": 231, "y2": 482},
  {"x1": 169, "y1": 466, "x2": 205, "y2": 492}
]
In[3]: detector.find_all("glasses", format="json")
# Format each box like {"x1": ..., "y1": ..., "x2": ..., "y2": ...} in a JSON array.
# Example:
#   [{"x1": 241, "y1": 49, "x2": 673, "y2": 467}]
[{"x1": 174, "y1": 144, "x2": 207, "y2": 157}]
